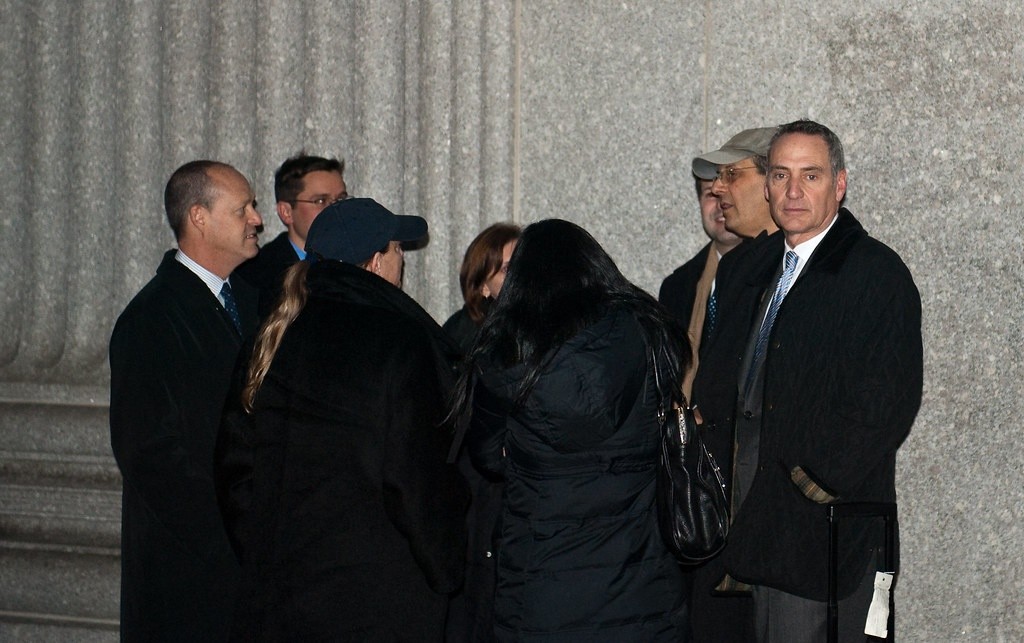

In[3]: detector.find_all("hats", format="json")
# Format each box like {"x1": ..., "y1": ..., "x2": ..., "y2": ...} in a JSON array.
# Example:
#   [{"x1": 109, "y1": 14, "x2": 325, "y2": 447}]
[
  {"x1": 692, "y1": 127, "x2": 780, "y2": 180},
  {"x1": 305, "y1": 197, "x2": 428, "y2": 265}
]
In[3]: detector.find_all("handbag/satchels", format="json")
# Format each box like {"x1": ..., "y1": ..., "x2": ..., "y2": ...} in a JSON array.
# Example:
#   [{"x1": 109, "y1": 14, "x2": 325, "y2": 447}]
[{"x1": 639, "y1": 305, "x2": 731, "y2": 562}]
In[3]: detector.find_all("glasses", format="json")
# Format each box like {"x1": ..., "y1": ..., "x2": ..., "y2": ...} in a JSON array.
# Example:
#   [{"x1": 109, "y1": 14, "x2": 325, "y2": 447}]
[
  {"x1": 283, "y1": 196, "x2": 353, "y2": 212},
  {"x1": 716, "y1": 165, "x2": 759, "y2": 185}
]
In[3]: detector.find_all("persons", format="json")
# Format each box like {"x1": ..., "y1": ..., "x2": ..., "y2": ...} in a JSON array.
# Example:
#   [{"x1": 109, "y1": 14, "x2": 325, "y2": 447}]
[{"x1": 108, "y1": 118, "x2": 924, "y2": 643}]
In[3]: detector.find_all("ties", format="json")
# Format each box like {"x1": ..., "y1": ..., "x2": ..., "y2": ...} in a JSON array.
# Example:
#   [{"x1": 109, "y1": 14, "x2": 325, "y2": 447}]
[
  {"x1": 747, "y1": 251, "x2": 799, "y2": 385},
  {"x1": 220, "y1": 282, "x2": 246, "y2": 343},
  {"x1": 702, "y1": 289, "x2": 716, "y2": 350}
]
